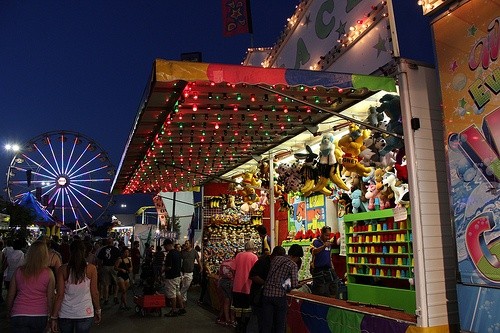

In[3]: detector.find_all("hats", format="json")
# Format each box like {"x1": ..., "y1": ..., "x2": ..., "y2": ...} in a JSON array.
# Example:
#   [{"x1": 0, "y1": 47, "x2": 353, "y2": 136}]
[{"x1": 161, "y1": 240, "x2": 173, "y2": 246}]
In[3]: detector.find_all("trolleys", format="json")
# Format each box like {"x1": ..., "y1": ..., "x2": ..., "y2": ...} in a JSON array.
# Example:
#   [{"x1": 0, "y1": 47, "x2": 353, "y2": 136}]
[{"x1": 124, "y1": 271, "x2": 165, "y2": 318}]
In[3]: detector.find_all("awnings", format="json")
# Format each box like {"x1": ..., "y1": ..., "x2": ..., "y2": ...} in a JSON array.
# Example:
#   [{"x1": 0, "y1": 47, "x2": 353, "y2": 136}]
[{"x1": 109, "y1": 59, "x2": 396, "y2": 195}]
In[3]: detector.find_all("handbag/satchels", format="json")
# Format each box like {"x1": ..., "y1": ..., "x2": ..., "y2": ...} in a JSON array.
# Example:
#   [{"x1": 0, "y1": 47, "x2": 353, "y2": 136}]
[{"x1": 254, "y1": 286, "x2": 264, "y2": 306}]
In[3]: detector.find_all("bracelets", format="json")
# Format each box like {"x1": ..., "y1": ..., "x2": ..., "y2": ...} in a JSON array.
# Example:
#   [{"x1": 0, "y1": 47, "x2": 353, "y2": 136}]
[
  {"x1": 95, "y1": 309, "x2": 102, "y2": 313},
  {"x1": 50, "y1": 316, "x2": 57, "y2": 319}
]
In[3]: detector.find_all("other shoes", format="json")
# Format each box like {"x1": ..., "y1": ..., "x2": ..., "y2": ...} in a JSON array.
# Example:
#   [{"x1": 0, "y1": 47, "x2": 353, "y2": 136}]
[
  {"x1": 216, "y1": 318, "x2": 237, "y2": 329},
  {"x1": 120, "y1": 305, "x2": 132, "y2": 312},
  {"x1": 165, "y1": 310, "x2": 179, "y2": 317},
  {"x1": 104, "y1": 300, "x2": 109, "y2": 305},
  {"x1": 113, "y1": 297, "x2": 120, "y2": 304},
  {"x1": 182, "y1": 309, "x2": 187, "y2": 313}
]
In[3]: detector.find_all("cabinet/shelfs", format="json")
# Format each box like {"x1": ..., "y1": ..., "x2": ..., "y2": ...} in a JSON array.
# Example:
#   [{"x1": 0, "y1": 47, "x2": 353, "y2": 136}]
[{"x1": 343, "y1": 207, "x2": 416, "y2": 314}]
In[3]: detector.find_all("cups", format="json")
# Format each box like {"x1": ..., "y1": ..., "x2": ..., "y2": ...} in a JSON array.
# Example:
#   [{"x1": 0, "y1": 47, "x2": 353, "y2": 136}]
[{"x1": 349, "y1": 222, "x2": 415, "y2": 279}]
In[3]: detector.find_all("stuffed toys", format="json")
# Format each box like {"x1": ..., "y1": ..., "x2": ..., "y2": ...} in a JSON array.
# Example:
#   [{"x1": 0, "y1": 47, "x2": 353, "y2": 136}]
[
  {"x1": 203, "y1": 197, "x2": 261, "y2": 278},
  {"x1": 224, "y1": 93, "x2": 411, "y2": 214}
]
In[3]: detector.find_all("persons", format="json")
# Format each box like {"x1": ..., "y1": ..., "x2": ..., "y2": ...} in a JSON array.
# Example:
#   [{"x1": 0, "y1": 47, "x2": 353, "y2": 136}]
[
  {"x1": 160, "y1": 239, "x2": 187, "y2": 317},
  {"x1": 309, "y1": 226, "x2": 341, "y2": 299},
  {"x1": 3, "y1": 241, "x2": 56, "y2": 333},
  {"x1": 230, "y1": 241, "x2": 259, "y2": 333},
  {"x1": 131, "y1": 239, "x2": 202, "y2": 290},
  {"x1": 281, "y1": 258, "x2": 302, "y2": 291},
  {"x1": 96, "y1": 237, "x2": 123, "y2": 305},
  {"x1": 0, "y1": 232, "x2": 133, "y2": 312},
  {"x1": 217, "y1": 250, "x2": 238, "y2": 326},
  {"x1": 259, "y1": 244, "x2": 313, "y2": 333},
  {"x1": 180, "y1": 240, "x2": 202, "y2": 305},
  {"x1": 114, "y1": 248, "x2": 132, "y2": 308},
  {"x1": 248, "y1": 246, "x2": 286, "y2": 333},
  {"x1": 50, "y1": 241, "x2": 102, "y2": 333}
]
